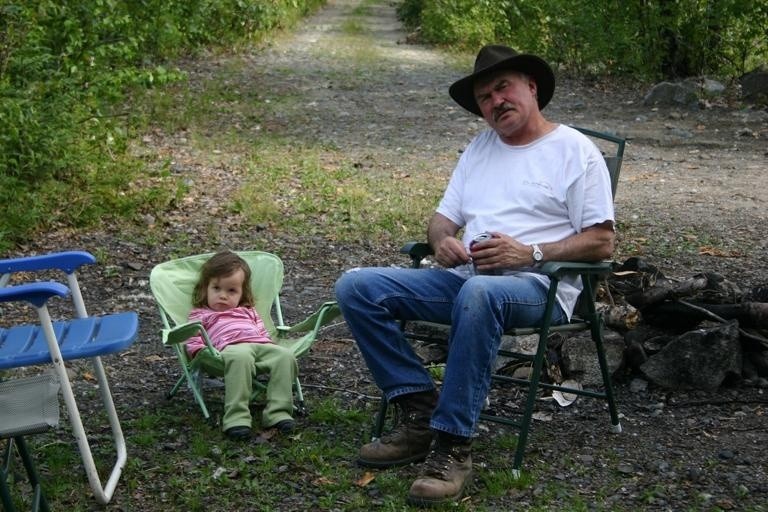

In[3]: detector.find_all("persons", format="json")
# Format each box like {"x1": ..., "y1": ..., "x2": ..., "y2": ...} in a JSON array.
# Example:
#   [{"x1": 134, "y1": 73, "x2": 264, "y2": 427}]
[
  {"x1": 334, "y1": 45, "x2": 617, "y2": 509},
  {"x1": 184, "y1": 251, "x2": 298, "y2": 440}
]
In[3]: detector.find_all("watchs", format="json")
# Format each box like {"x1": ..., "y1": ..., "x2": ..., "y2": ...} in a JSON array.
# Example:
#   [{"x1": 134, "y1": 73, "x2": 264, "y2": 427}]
[{"x1": 530, "y1": 244, "x2": 544, "y2": 268}]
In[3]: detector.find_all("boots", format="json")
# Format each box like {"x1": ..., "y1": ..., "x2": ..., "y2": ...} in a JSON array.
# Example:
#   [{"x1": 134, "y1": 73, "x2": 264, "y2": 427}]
[
  {"x1": 357, "y1": 388, "x2": 441, "y2": 467},
  {"x1": 408, "y1": 432, "x2": 474, "y2": 505}
]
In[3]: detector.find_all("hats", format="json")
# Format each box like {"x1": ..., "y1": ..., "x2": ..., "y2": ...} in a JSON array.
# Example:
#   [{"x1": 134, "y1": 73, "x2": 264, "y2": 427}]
[{"x1": 448, "y1": 45, "x2": 555, "y2": 116}]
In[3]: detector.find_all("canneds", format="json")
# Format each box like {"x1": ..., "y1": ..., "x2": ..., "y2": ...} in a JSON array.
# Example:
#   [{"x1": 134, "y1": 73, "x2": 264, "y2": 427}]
[{"x1": 470, "y1": 234, "x2": 502, "y2": 276}]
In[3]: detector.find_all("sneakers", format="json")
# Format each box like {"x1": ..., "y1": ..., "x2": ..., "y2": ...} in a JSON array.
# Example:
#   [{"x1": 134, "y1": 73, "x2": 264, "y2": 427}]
[
  {"x1": 226, "y1": 427, "x2": 251, "y2": 441},
  {"x1": 274, "y1": 420, "x2": 295, "y2": 431}
]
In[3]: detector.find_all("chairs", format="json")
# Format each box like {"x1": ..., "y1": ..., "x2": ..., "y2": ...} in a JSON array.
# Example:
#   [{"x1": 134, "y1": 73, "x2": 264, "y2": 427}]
[
  {"x1": 1, "y1": 251, "x2": 138, "y2": 511},
  {"x1": 370, "y1": 126, "x2": 625, "y2": 477},
  {"x1": 149, "y1": 249, "x2": 343, "y2": 420}
]
